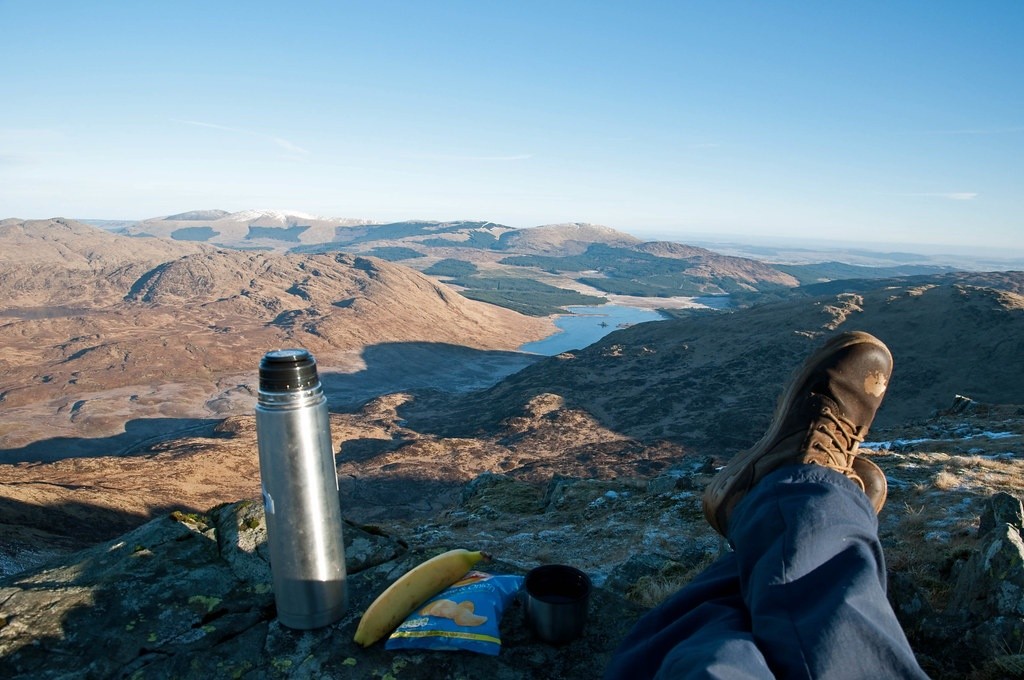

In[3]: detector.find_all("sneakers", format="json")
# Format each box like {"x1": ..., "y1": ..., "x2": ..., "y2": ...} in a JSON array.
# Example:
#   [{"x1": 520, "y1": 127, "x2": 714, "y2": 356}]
[
  {"x1": 844, "y1": 455, "x2": 889, "y2": 516},
  {"x1": 701, "y1": 330, "x2": 894, "y2": 543}
]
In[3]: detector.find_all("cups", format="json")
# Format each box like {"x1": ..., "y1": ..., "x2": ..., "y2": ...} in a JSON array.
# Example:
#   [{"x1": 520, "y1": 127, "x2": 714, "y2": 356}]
[{"x1": 524, "y1": 565, "x2": 593, "y2": 642}]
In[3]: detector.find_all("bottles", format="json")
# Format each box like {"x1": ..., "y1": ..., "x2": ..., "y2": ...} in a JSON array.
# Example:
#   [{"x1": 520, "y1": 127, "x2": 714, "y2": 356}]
[{"x1": 256, "y1": 349, "x2": 349, "y2": 629}]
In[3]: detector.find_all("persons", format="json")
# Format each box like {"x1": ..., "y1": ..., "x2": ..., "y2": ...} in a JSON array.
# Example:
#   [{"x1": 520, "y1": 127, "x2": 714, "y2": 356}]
[{"x1": 599, "y1": 329, "x2": 934, "y2": 680}]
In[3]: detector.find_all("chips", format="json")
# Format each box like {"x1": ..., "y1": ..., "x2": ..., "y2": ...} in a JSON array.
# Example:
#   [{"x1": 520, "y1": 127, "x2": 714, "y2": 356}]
[{"x1": 418, "y1": 599, "x2": 489, "y2": 626}]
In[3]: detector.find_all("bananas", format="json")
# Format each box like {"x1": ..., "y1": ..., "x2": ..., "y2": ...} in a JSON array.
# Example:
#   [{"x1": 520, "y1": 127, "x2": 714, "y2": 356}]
[{"x1": 354, "y1": 549, "x2": 486, "y2": 646}]
